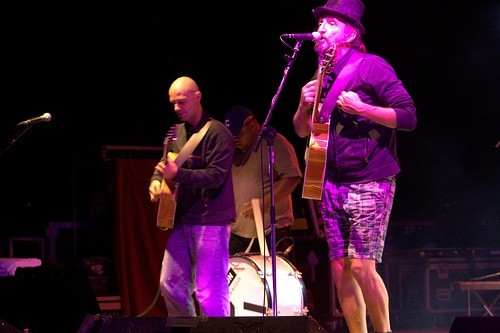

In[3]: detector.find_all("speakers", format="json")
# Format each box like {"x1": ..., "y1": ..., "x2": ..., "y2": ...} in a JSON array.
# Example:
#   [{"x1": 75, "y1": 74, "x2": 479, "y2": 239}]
[
  {"x1": 449, "y1": 316, "x2": 500, "y2": 333},
  {"x1": 78, "y1": 314, "x2": 329, "y2": 333}
]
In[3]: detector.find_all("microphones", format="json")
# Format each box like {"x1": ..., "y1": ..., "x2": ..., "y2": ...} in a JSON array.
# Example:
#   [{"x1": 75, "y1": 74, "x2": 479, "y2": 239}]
[
  {"x1": 18, "y1": 113, "x2": 51, "y2": 126},
  {"x1": 282, "y1": 32, "x2": 321, "y2": 42}
]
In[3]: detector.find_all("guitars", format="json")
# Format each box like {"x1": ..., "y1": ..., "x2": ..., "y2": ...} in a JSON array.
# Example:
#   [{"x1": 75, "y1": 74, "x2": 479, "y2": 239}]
[
  {"x1": 301, "y1": 42, "x2": 339, "y2": 201},
  {"x1": 156, "y1": 125, "x2": 180, "y2": 231}
]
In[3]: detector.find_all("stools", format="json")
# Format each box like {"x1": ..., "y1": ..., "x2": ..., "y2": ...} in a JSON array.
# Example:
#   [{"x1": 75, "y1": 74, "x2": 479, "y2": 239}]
[{"x1": 451, "y1": 282, "x2": 500, "y2": 316}]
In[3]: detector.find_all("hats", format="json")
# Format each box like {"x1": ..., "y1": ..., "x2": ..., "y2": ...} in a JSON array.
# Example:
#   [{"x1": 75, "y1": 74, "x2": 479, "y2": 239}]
[
  {"x1": 225, "y1": 107, "x2": 252, "y2": 135},
  {"x1": 312, "y1": 0, "x2": 365, "y2": 35}
]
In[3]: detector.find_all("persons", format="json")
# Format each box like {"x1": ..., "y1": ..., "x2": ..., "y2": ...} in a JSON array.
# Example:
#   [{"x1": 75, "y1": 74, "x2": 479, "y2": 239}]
[
  {"x1": 148, "y1": 77, "x2": 236, "y2": 317},
  {"x1": 225, "y1": 108, "x2": 304, "y2": 261},
  {"x1": 292, "y1": 0, "x2": 417, "y2": 333}
]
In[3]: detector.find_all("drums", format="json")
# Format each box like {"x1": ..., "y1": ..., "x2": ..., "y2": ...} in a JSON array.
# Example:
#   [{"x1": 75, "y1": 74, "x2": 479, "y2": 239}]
[{"x1": 226, "y1": 252, "x2": 308, "y2": 318}]
